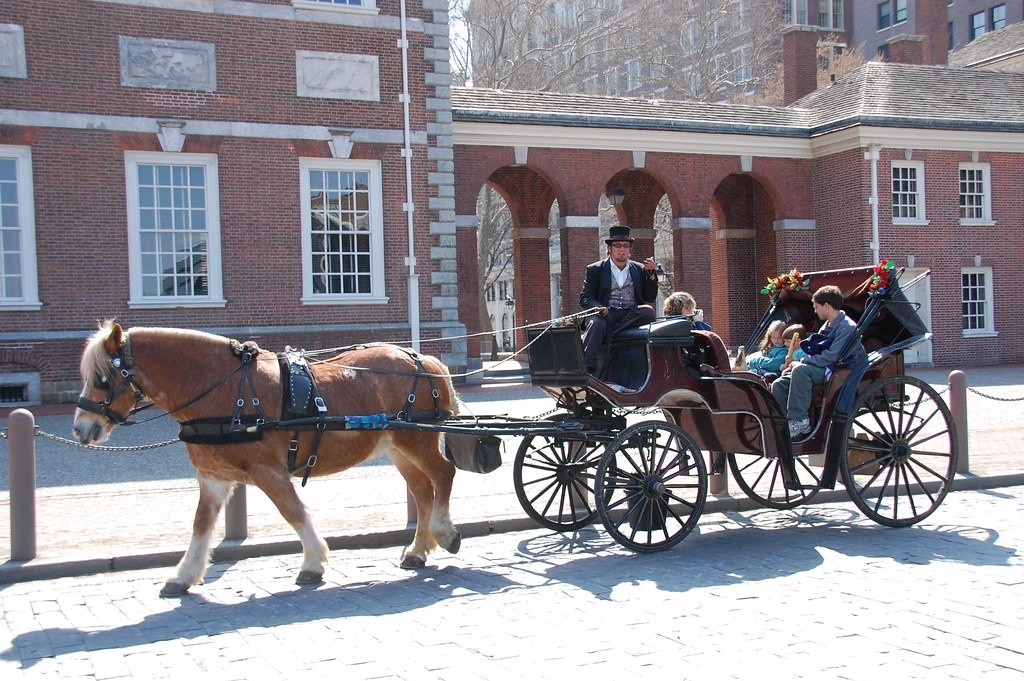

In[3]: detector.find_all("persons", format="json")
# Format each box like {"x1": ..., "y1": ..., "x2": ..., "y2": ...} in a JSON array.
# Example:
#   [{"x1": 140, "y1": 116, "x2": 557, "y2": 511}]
[
  {"x1": 663, "y1": 291, "x2": 713, "y2": 331},
  {"x1": 579, "y1": 226, "x2": 658, "y2": 375},
  {"x1": 746, "y1": 286, "x2": 866, "y2": 437}
]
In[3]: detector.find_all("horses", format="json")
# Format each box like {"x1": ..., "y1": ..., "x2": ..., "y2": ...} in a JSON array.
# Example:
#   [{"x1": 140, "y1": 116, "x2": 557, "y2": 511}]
[{"x1": 71, "y1": 317, "x2": 467, "y2": 599}]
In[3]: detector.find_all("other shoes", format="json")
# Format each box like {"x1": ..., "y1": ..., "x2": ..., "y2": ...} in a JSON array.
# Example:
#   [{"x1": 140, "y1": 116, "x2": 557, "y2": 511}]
[{"x1": 586, "y1": 367, "x2": 595, "y2": 374}]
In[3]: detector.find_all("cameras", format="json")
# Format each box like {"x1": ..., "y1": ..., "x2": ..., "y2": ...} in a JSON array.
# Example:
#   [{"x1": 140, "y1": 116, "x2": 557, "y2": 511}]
[{"x1": 690, "y1": 311, "x2": 700, "y2": 321}]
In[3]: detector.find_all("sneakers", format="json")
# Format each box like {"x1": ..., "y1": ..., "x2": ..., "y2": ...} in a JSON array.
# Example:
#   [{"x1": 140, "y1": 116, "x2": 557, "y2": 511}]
[{"x1": 788, "y1": 417, "x2": 811, "y2": 437}]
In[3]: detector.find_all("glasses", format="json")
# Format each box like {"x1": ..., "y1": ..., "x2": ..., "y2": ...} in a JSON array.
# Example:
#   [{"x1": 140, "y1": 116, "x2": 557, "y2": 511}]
[
  {"x1": 611, "y1": 243, "x2": 630, "y2": 249},
  {"x1": 813, "y1": 304, "x2": 822, "y2": 310},
  {"x1": 770, "y1": 338, "x2": 782, "y2": 342}
]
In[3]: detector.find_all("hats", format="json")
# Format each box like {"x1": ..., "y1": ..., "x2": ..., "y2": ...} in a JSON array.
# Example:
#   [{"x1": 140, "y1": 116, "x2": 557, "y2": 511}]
[{"x1": 605, "y1": 226, "x2": 635, "y2": 245}]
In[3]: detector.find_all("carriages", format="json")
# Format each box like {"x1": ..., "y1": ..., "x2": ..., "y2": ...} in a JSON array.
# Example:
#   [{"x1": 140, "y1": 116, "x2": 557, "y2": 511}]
[{"x1": 72, "y1": 260, "x2": 960, "y2": 598}]
